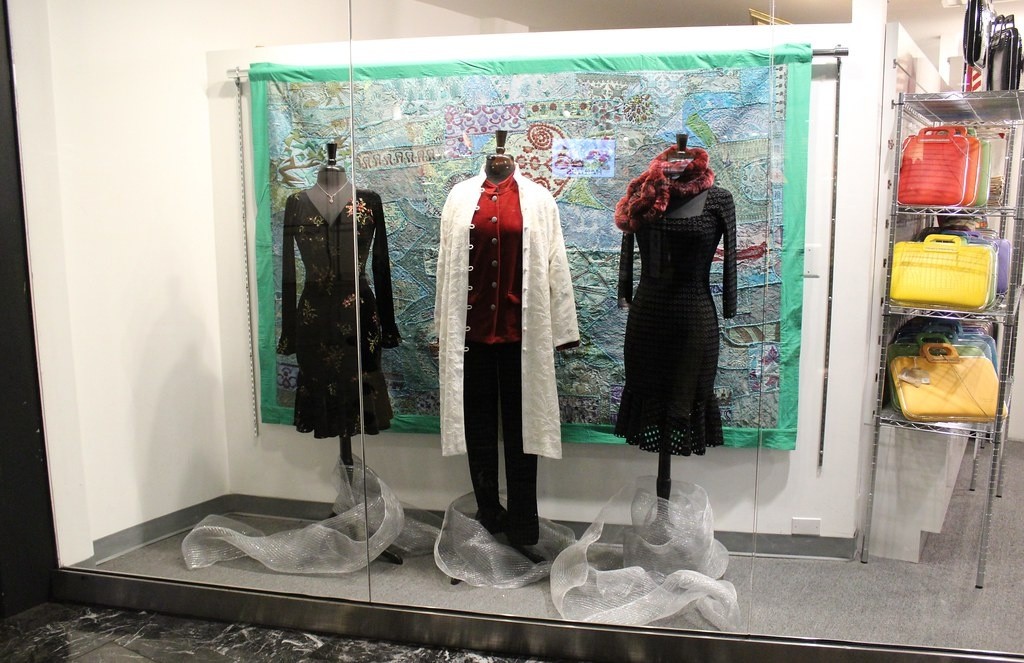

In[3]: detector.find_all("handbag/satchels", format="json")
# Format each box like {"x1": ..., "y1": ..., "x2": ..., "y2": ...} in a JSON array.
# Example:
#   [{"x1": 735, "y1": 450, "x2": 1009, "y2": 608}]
[
  {"x1": 898, "y1": 122, "x2": 992, "y2": 207},
  {"x1": 889, "y1": 212, "x2": 1012, "y2": 311},
  {"x1": 885, "y1": 317, "x2": 1008, "y2": 421}
]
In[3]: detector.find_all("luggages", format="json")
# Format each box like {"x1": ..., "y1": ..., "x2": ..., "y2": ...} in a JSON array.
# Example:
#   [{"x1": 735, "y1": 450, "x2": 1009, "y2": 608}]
[{"x1": 979, "y1": 14, "x2": 1023, "y2": 91}]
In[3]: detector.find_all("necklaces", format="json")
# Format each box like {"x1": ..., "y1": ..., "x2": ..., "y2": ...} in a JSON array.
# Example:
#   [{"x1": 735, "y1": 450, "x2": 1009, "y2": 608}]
[{"x1": 316, "y1": 177, "x2": 348, "y2": 204}]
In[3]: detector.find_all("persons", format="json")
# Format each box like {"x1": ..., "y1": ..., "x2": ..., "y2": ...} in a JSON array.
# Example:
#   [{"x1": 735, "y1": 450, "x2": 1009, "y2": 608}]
[
  {"x1": 620, "y1": 145, "x2": 738, "y2": 457},
  {"x1": 438, "y1": 157, "x2": 581, "y2": 563},
  {"x1": 276, "y1": 165, "x2": 400, "y2": 438}
]
